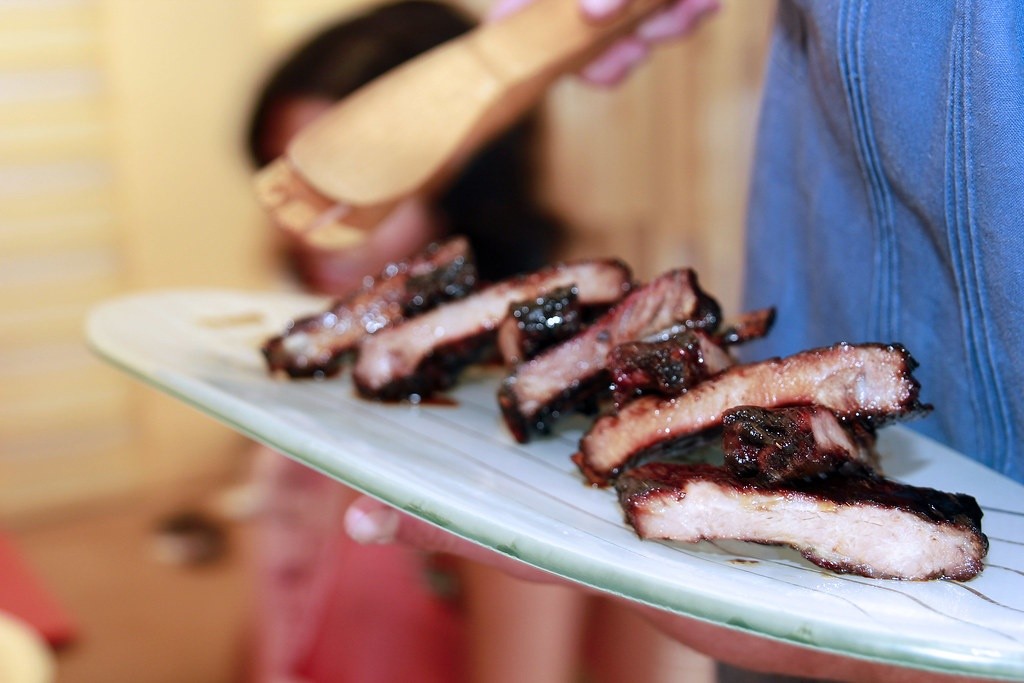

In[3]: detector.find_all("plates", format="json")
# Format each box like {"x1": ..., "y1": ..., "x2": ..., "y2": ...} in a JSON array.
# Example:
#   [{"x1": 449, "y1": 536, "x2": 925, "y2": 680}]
[{"x1": 85, "y1": 287, "x2": 1024, "y2": 683}]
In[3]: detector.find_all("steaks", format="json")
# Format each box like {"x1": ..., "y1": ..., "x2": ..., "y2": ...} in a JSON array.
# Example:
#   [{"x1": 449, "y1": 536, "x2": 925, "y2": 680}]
[{"x1": 260, "y1": 235, "x2": 990, "y2": 579}]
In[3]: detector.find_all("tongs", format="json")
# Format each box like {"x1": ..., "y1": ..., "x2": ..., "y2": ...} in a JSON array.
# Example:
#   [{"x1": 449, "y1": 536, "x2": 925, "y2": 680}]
[{"x1": 251, "y1": 0, "x2": 685, "y2": 255}]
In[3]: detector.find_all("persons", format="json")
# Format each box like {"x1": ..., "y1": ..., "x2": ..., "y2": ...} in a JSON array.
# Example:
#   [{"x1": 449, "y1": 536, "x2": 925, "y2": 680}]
[
  {"x1": 226, "y1": 2, "x2": 715, "y2": 683},
  {"x1": 342, "y1": 1, "x2": 1022, "y2": 683}
]
